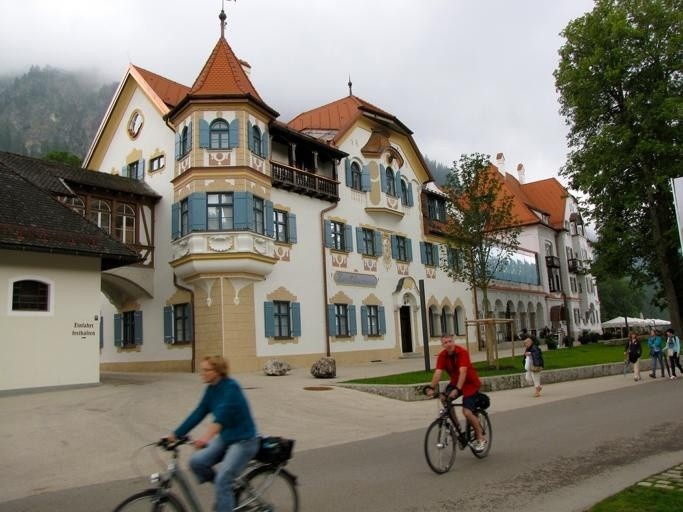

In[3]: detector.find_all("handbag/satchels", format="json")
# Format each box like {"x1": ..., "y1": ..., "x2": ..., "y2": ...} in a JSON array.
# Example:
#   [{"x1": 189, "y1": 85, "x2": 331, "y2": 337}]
[
  {"x1": 476, "y1": 393, "x2": 489, "y2": 409},
  {"x1": 531, "y1": 365, "x2": 541, "y2": 372},
  {"x1": 254, "y1": 436, "x2": 293, "y2": 463}
]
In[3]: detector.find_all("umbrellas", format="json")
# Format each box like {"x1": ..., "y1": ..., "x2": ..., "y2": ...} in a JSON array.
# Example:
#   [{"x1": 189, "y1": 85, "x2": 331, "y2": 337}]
[
  {"x1": 631, "y1": 318, "x2": 672, "y2": 328},
  {"x1": 623, "y1": 352, "x2": 630, "y2": 379},
  {"x1": 663, "y1": 351, "x2": 671, "y2": 377}
]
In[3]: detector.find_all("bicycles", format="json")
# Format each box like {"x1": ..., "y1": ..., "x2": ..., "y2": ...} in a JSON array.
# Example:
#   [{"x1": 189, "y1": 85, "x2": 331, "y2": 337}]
[
  {"x1": 114, "y1": 437, "x2": 299, "y2": 512},
  {"x1": 424, "y1": 389, "x2": 492, "y2": 474}
]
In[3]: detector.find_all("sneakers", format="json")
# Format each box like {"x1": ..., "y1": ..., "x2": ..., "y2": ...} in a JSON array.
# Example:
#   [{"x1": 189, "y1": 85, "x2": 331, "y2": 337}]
[
  {"x1": 649, "y1": 374, "x2": 677, "y2": 379},
  {"x1": 532, "y1": 387, "x2": 542, "y2": 397},
  {"x1": 634, "y1": 377, "x2": 641, "y2": 381},
  {"x1": 476, "y1": 439, "x2": 488, "y2": 451}
]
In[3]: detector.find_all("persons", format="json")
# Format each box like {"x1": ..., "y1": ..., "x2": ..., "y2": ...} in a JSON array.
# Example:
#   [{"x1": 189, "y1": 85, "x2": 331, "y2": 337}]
[
  {"x1": 420, "y1": 333, "x2": 489, "y2": 453},
  {"x1": 647, "y1": 329, "x2": 665, "y2": 377},
  {"x1": 158, "y1": 352, "x2": 260, "y2": 512},
  {"x1": 521, "y1": 333, "x2": 546, "y2": 398},
  {"x1": 623, "y1": 331, "x2": 644, "y2": 382},
  {"x1": 660, "y1": 328, "x2": 682, "y2": 380}
]
